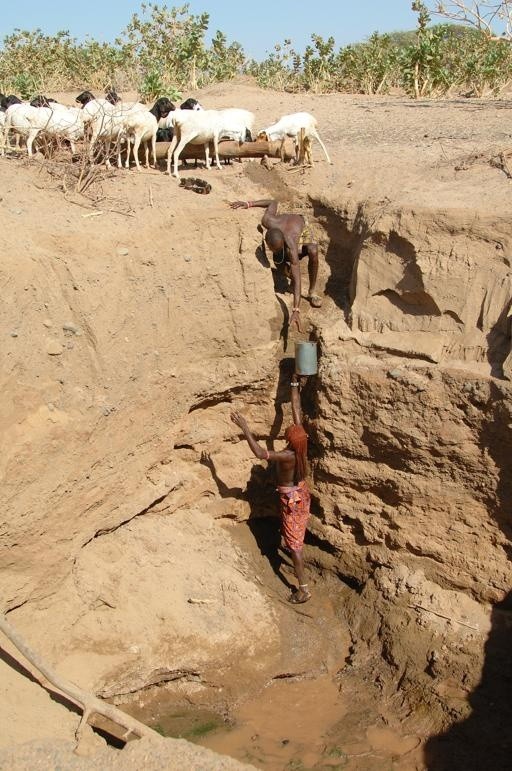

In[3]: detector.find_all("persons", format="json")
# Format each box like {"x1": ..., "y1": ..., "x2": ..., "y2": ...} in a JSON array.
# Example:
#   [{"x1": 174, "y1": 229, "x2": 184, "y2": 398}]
[
  {"x1": 229, "y1": 199, "x2": 322, "y2": 332},
  {"x1": 232, "y1": 373, "x2": 312, "y2": 604}
]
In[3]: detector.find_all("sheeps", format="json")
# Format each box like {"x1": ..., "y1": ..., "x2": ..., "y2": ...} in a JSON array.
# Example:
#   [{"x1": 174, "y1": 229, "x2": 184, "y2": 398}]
[
  {"x1": 0, "y1": 91, "x2": 257, "y2": 179},
  {"x1": 256, "y1": 112, "x2": 331, "y2": 164}
]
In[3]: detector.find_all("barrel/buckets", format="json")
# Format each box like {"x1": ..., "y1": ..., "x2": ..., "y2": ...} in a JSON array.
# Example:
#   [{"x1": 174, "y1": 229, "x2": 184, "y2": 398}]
[{"x1": 294, "y1": 340, "x2": 319, "y2": 376}]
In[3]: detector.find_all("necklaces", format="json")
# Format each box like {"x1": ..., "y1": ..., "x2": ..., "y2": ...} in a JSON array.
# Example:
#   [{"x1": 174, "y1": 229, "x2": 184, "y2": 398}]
[{"x1": 273, "y1": 251, "x2": 285, "y2": 266}]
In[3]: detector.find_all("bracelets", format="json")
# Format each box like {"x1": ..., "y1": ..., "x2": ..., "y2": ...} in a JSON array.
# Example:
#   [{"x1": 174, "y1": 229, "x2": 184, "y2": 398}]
[
  {"x1": 247, "y1": 200, "x2": 255, "y2": 207},
  {"x1": 291, "y1": 307, "x2": 300, "y2": 312},
  {"x1": 290, "y1": 382, "x2": 301, "y2": 388}
]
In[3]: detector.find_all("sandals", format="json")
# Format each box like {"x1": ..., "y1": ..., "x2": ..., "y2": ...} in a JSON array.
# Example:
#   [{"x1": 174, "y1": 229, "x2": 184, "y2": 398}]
[
  {"x1": 287, "y1": 584, "x2": 311, "y2": 603},
  {"x1": 307, "y1": 294, "x2": 323, "y2": 307}
]
